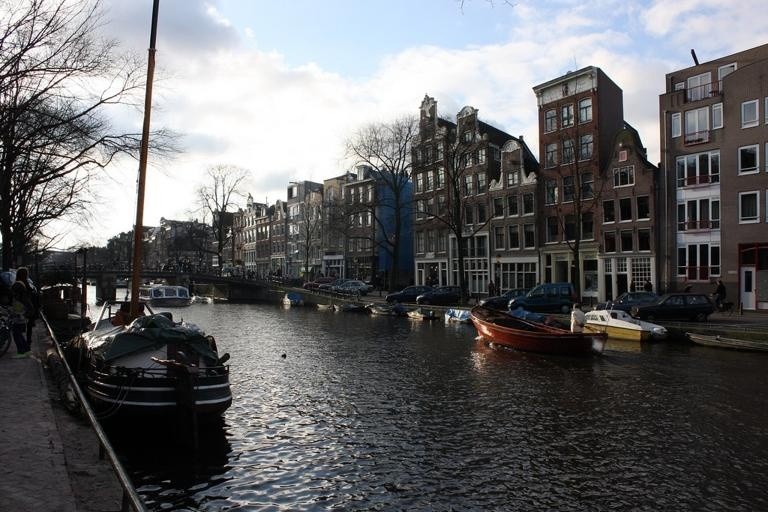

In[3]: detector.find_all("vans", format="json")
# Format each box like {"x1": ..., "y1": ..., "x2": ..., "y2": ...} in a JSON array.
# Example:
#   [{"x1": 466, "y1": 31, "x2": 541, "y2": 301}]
[{"x1": 508, "y1": 283, "x2": 578, "y2": 314}]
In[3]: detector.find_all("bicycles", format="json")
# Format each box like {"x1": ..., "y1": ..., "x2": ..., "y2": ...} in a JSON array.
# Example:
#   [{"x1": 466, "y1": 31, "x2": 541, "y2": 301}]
[
  {"x1": 708, "y1": 294, "x2": 734, "y2": 318},
  {"x1": 0, "y1": 304, "x2": 15, "y2": 358}
]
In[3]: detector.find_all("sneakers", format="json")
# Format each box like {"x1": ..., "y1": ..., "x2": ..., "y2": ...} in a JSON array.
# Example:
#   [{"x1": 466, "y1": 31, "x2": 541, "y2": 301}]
[{"x1": 14, "y1": 351, "x2": 35, "y2": 359}]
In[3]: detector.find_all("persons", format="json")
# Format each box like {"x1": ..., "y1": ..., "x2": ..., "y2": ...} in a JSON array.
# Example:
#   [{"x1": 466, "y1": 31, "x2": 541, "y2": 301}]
[
  {"x1": 10, "y1": 267, "x2": 34, "y2": 359},
  {"x1": 630, "y1": 283, "x2": 635, "y2": 292},
  {"x1": 7, "y1": 268, "x2": 38, "y2": 346},
  {"x1": 488, "y1": 280, "x2": 495, "y2": 297},
  {"x1": 569, "y1": 302, "x2": 586, "y2": 333},
  {"x1": 148, "y1": 350, "x2": 196, "y2": 426},
  {"x1": 643, "y1": 281, "x2": 652, "y2": 292},
  {"x1": 390, "y1": 300, "x2": 409, "y2": 318},
  {"x1": 709, "y1": 279, "x2": 726, "y2": 313}
]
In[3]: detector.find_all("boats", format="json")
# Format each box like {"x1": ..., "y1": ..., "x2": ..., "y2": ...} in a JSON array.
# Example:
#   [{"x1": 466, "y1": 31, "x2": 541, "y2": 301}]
[
  {"x1": 279, "y1": 293, "x2": 305, "y2": 306},
  {"x1": 684, "y1": 332, "x2": 768, "y2": 351}
]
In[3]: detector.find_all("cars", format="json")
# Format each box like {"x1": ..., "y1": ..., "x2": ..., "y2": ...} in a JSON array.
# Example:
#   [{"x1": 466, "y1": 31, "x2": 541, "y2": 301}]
[
  {"x1": 479, "y1": 288, "x2": 530, "y2": 311},
  {"x1": 593, "y1": 292, "x2": 714, "y2": 322},
  {"x1": 303, "y1": 277, "x2": 374, "y2": 296},
  {"x1": 385, "y1": 285, "x2": 470, "y2": 304}
]
